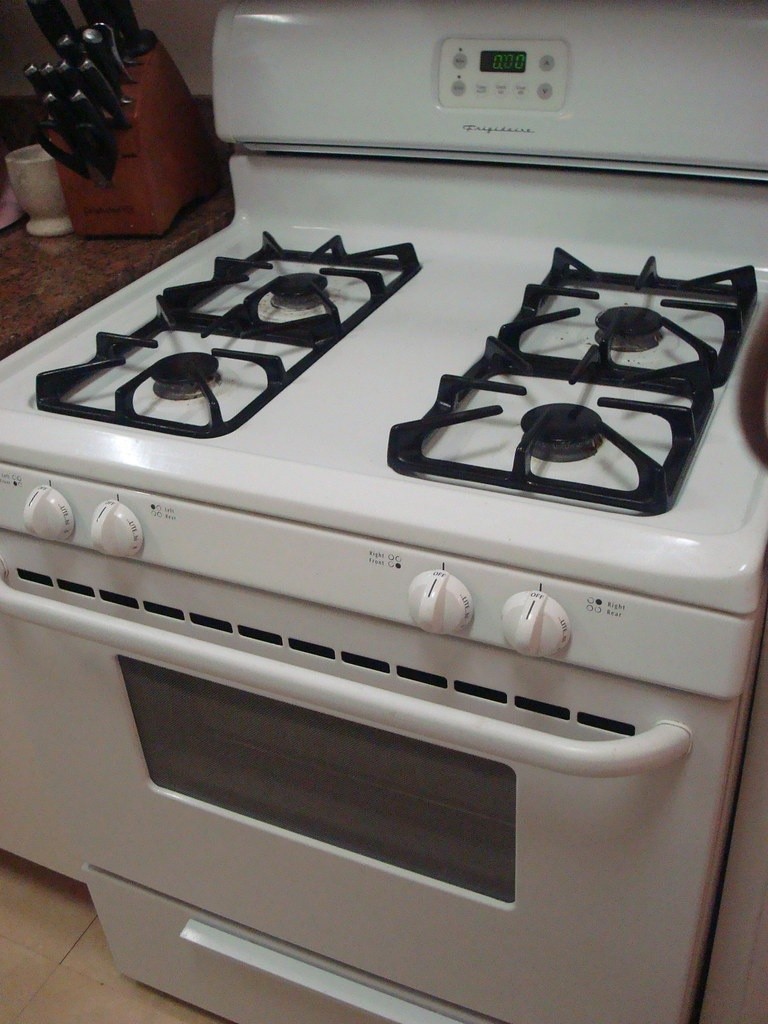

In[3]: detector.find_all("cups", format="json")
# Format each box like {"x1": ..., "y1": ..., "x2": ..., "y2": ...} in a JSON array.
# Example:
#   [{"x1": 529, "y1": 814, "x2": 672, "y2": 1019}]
[{"x1": 5, "y1": 144, "x2": 75, "y2": 236}]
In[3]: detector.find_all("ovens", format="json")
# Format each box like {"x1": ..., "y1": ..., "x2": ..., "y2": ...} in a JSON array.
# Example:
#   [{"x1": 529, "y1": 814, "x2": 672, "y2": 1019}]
[{"x1": 0, "y1": 530, "x2": 754, "y2": 1024}]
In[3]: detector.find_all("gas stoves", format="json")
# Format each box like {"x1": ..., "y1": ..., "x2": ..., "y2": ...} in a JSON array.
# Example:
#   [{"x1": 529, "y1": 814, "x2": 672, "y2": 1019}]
[{"x1": 1, "y1": 0, "x2": 767, "y2": 702}]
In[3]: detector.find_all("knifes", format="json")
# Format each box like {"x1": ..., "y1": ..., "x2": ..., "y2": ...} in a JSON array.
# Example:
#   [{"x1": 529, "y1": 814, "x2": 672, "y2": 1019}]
[{"x1": 23, "y1": 1, "x2": 153, "y2": 158}]
